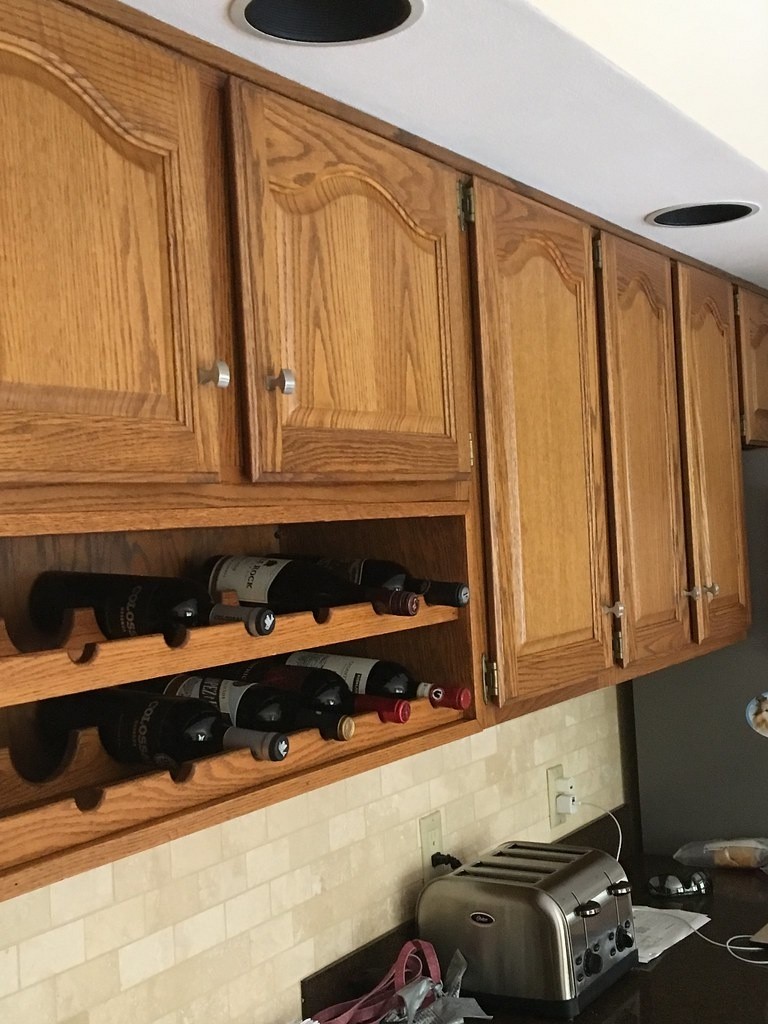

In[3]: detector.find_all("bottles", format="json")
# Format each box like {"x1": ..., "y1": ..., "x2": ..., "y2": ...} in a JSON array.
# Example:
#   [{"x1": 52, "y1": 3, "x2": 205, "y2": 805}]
[
  {"x1": 197, "y1": 554, "x2": 420, "y2": 616},
  {"x1": 274, "y1": 651, "x2": 471, "y2": 711},
  {"x1": 35, "y1": 687, "x2": 289, "y2": 768},
  {"x1": 155, "y1": 672, "x2": 356, "y2": 742},
  {"x1": 300, "y1": 553, "x2": 470, "y2": 607},
  {"x1": 18, "y1": 569, "x2": 276, "y2": 637},
  {"x1": 212, "y1": 662, "x2": 411, "y2": 723}
]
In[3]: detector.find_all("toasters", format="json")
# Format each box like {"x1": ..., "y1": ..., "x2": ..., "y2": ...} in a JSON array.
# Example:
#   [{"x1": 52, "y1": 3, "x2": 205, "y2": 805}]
[{"x1": 414, "y1": 841, "x2": 639, "y2": 1022}]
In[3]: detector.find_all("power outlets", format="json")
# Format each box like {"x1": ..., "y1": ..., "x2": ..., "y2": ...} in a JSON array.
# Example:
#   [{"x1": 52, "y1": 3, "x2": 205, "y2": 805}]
[
  {"x1": 419, "y1": 810, "x2": 446, "y2": 884},
  {"x1": 547, "y1": 764, "x2": 567, "y2": 828}
]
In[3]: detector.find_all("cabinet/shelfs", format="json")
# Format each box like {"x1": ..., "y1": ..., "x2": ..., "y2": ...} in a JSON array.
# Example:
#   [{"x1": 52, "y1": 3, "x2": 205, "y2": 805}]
[
  {"x1": 0, "y1": 0, "x2": 491, "y2": 902},
  {"x1": 595, "y1": 225, "x2": 768, "y2": 682},
  {"x1": 465, "y1": 175, "x2": 623, "y2": 724}
]
